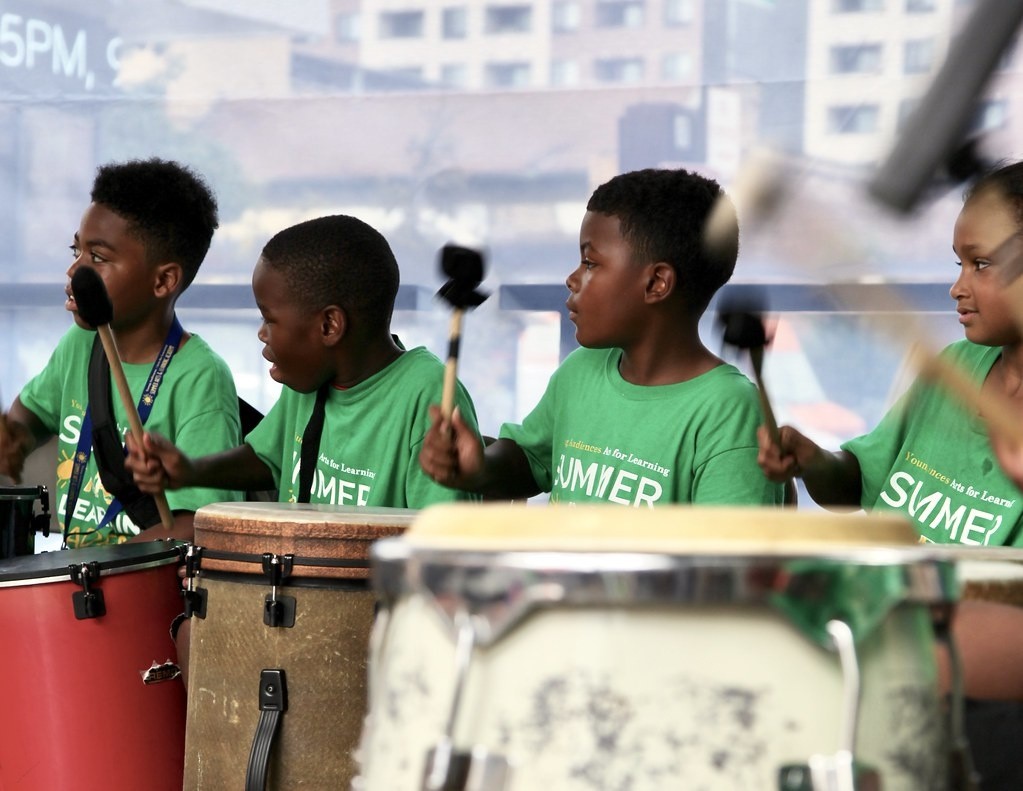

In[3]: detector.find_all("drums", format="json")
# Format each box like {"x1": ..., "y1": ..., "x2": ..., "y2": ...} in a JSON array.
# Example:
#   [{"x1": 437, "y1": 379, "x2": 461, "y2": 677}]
[{"x1": 2, "y1": 500, "x2": 1022, "y2": 790}]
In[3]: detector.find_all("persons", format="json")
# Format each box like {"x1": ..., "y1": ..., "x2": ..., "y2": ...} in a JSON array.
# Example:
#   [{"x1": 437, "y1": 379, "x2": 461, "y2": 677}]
[
  {"x1": 124, "y1": 214, "x2": 487, "y2": 696},
  {"x1": 0, "y1": 157, "x2": 246, "y2": 543},
  {"x1": 758, "y1": 160, "x2": 1023, "y2": 701},
  {"x1": 418, "y1": 168, "x2": 786, "y2": 506}
]
